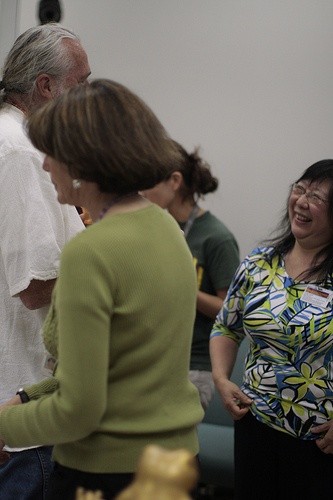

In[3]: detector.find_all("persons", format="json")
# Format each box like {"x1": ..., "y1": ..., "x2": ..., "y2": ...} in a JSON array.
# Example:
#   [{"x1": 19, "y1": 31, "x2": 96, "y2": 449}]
[
  {"x1": 0, "y1": 77, "x2": 204, "y2": 500},
  {"x1": 0, "y1": 24, "x2": 91, "y2": 500},
  {"x1": 140, "y1": 139, "x2": 240, "y2": 409},
  {"x1": 209, "y1": 158, "x2": 333, "y2": 500}
]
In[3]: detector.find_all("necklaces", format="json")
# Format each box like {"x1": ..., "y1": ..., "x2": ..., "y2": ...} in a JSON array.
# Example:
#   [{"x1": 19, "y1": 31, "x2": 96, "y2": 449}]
[{"x1": 94, "y1": 193, "x2": 128, "y2": 222}]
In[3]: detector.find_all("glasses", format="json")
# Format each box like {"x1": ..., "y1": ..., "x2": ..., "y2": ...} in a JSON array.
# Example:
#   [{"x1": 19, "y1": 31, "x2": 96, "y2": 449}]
[{"x1": 290, "y1": 182, "x2": 329, "y2": 206}]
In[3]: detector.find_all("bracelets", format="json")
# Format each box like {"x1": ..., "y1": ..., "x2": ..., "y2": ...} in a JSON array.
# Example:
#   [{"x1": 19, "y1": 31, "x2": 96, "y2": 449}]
[{"x1": 16, "y1": 389, "x2": 30, "y2": 403}]
